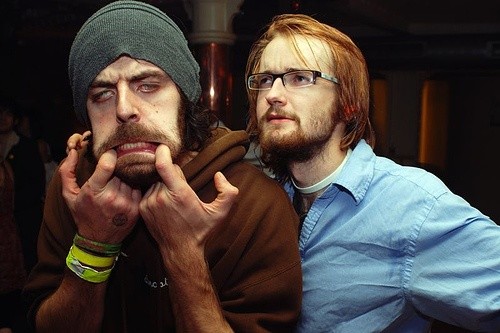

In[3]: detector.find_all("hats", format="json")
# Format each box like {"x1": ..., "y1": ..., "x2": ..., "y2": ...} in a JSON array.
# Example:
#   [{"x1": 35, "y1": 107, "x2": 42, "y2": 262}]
[{"x1": 68, "y1": 1, "x2": 202, "y2": 125}]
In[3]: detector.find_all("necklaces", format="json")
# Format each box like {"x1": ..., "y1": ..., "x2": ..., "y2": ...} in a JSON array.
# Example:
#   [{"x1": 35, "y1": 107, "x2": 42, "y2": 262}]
[{"x1": 290, "y1": 147, "x2": 353, "y2": 193}]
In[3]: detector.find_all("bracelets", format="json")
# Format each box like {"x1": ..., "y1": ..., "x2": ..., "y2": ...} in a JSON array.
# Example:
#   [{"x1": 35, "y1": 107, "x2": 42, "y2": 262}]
[{"x1": 65, "y1": 232, "x2": 121, "y2": 284}]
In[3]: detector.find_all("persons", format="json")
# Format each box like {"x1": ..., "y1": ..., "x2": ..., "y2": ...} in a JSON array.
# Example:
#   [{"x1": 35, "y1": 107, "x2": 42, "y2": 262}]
[
  {"x1": 7, "y1": 1, "x2": 302, "y2": 333},
  {"x1": 244, "y1": 15, "x2": 500, "y2": 333},
  {"x1": 0, "y1": 107, "x2": 60, "y2": 333}
]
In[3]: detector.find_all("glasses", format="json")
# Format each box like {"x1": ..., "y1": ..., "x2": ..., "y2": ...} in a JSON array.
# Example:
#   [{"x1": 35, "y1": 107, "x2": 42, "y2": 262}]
[{"x1": 247, "y1": 70, "x2": 340, "y2": 91}]
